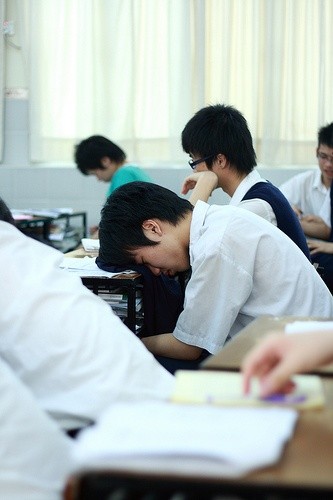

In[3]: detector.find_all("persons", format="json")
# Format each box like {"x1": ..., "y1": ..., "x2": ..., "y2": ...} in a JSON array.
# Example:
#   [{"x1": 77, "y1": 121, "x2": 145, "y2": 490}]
[
  {"x1": 307, "y1": 240, "x2": 333, "y2": 255},
  {"x1": 181, "y1": 103, "x2": 311, "y2": 263},
  {"x1": 0, "y1": 218, "x2": 175, "y2": 441},
  {"x1": 75, "y1": 134, "x2": 156, "y2": 202},
  {"x1": 0, "y1": 356, "x2": 77, "y2": 500},
  {"x1": 95, "y1": 182, "x2": 333, "y2": 363},
  {"x1": 278, "y1": 121, "x2": 333, "y2": 228},
  {"x1": 242, "y1": 321, "x2": 333, "y2": 400}
]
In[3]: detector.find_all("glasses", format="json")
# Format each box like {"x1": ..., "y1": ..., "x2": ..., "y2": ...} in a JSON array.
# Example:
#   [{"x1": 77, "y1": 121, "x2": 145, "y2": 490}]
[{"x1": 188, "y1": 153, "x2": 215, "y2": 169}]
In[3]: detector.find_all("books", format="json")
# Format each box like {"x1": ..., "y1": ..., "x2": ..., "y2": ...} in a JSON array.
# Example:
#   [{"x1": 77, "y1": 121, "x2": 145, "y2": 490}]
[
  {"x1": 68, "y1": 372, "x2": 323, "y2": 479},
  {"x1": 92, "y1": 289, "x2": 144, "y2": 319}
]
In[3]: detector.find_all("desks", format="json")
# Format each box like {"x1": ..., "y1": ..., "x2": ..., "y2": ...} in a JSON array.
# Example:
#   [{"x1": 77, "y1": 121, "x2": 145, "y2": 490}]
[{"x1": 9, "y1": 205, "x2": 333, "y2": 500}]
